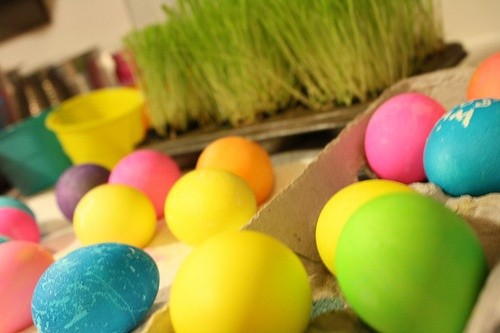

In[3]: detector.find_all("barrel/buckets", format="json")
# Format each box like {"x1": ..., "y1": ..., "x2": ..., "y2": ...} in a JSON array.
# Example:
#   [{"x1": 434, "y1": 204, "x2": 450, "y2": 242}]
[
  {"x1": 0, "y1": 110, "x2": 70, "y2": 194},
  {"x1": 46, "y1": 86, "x2": 148, "y2": 166}
]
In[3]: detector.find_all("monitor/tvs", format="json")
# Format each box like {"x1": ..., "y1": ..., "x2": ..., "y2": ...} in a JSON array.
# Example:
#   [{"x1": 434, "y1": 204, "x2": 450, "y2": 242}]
[{"x1": 0, "y1": 0, "x2": 49, "y2": 42}]
